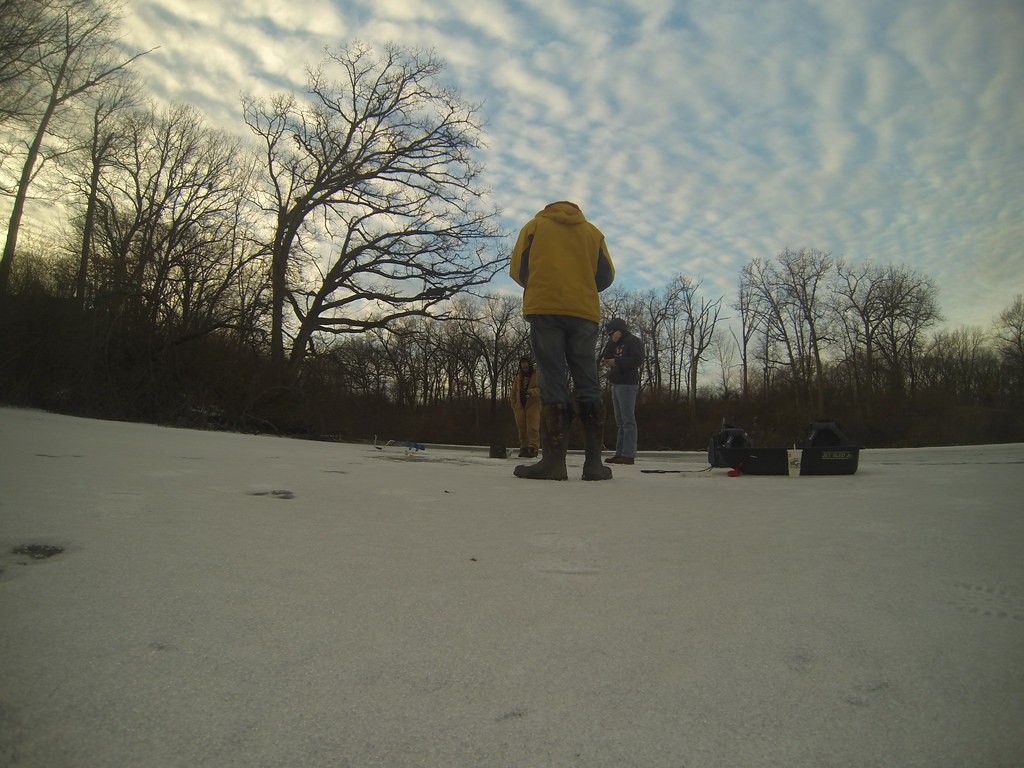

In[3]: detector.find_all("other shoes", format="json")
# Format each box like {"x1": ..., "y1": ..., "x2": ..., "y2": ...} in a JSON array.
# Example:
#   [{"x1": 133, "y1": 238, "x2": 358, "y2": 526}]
[
  {"x1": 518, "y1": 445, "x2": 539, "y2": 458},
  {"x1": 605, "y1": 454, "x2": 635, "y2": 465}
]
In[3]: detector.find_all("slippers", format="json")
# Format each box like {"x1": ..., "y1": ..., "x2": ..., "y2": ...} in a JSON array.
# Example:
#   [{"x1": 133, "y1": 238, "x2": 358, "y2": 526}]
[{"x1": 513, "y1": 402, "x2": 576, "y2": 481}]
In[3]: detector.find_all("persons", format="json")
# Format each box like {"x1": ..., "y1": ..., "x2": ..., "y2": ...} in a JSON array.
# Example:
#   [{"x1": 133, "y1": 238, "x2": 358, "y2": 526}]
[
  {"x1": 604, "y1": 318, "x2": 645, "y2": 465},
  {"x1": 509, "y1": 201, "x2": 613, "y2": 480},
  {"x1": 510, "y1": 357, "x2": 542, "y2": 458}
]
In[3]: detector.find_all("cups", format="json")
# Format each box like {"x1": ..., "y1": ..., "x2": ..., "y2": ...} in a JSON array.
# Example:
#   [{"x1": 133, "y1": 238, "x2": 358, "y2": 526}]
[{"x1": 786, "y1": 449, "x2": 803, "y2": 478}]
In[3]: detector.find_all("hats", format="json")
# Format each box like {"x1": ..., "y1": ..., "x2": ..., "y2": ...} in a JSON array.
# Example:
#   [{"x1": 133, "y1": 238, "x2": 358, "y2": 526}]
[{"x1": 607, "y1": 326, "x2": 616, "y2": 335}]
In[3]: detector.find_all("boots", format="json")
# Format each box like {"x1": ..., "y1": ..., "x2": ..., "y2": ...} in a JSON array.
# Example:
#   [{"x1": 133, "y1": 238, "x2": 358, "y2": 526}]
[{"x1": 582, "y1": 400, "x2": 613, "y2": 481}]
